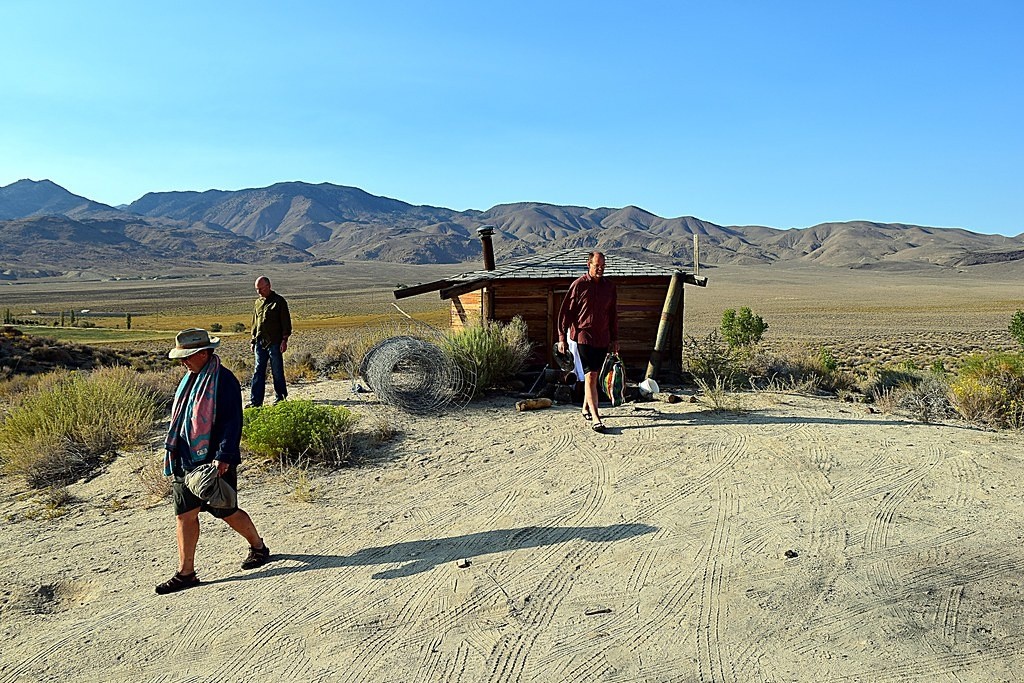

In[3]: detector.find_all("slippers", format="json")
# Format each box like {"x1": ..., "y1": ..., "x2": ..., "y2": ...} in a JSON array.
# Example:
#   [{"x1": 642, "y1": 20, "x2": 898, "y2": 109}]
[
  {"x1": 581, "y1": 410, "x2": 593, "y2": 420},
  {"x1": 591, "y1": 422, "x2": 607, "y2": 432}
]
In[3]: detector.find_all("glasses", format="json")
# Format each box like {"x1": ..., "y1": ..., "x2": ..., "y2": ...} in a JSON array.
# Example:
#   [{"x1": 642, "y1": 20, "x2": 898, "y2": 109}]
[
  {"x1": 590, "y1": 262, "x2": 607, "y2": 268},
  {"x1": 180, "y1": 354, "x2": 194, "y2": 360}
]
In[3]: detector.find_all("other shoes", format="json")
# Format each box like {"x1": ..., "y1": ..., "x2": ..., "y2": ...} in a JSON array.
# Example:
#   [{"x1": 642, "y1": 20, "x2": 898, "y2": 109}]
[
  {"x1": 274, "y1": 394, "x2": 288, "y2": 405},
  {"x1": 244, "y1": 402, "x2": 262, "y2": 408}
]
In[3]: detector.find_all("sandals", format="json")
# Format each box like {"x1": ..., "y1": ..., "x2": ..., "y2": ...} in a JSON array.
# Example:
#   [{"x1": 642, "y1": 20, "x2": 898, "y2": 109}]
[
  {"x1": 155, "y1": 570, "x2": 200, "y2": 594},
  {"x1": 241, "y1": 538, "x2": 271, "y2": 570}
]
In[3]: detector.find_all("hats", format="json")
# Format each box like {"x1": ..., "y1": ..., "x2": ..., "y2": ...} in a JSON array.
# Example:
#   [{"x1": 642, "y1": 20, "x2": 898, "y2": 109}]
[{"x1": 168, "y1": 328, "x2": 221, "y2": 358}]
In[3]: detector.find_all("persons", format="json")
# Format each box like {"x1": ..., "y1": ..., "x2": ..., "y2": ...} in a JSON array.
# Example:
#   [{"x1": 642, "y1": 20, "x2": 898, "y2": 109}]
[
  {"x1": 156, "y1": 327, "x2": 270, "y2": 594},
  {"x1": 244, "y1": 275, "x2": 292, "y2": 409},
  {"x1": 558, "y1": 252, "x2": 619, "y2": 431}
]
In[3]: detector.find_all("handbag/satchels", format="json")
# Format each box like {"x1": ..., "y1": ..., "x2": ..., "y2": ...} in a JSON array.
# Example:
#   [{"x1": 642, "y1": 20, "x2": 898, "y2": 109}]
[
  {"x1": 185, "y1": 464, "x2": 236, "y2": 509},
  {"x1": 598, "y1": 353, "x2": 626, "y2": 407}
]
